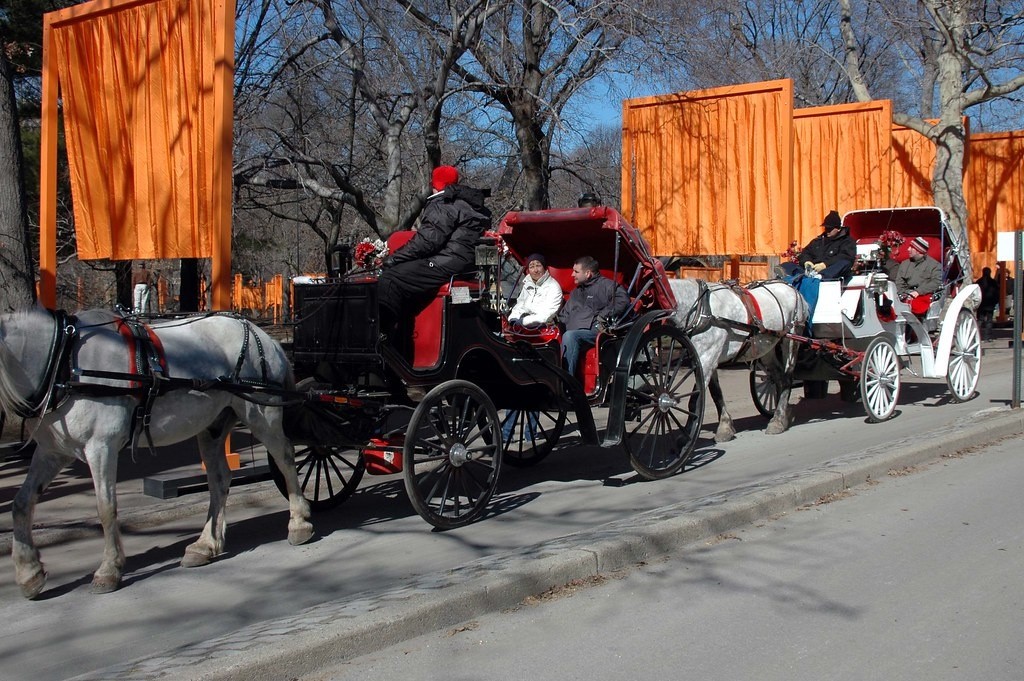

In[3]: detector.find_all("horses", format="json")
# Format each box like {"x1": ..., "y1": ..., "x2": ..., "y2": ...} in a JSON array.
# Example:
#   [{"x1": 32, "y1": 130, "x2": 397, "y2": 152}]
[
  {"x1": 668, "y1": 277, "x2": 809, "y2": 443},
  {"x1": 0, "y1": 302, "x2": 311, "y2": 598}
]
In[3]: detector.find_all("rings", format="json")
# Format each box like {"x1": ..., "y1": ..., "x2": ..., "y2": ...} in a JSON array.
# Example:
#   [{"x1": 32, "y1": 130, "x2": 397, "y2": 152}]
[{"x1": 913, "y1": 295, "x2": 915, "y2": 297}]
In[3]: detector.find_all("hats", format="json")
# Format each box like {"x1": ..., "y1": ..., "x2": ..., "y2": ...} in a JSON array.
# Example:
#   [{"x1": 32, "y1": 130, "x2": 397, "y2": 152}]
[
  {"x1": 527, "y1": 253, "x2": 547, "y2": 270},
  {"x1": 910, "y1": 236, "x2": 929, "y2": 254},
  {"x1": 432, "y1": 166, "x2": 459, "y2": 191},
  {"x1": 820, "y1": 211, "x2": 841, "y2": 228}
]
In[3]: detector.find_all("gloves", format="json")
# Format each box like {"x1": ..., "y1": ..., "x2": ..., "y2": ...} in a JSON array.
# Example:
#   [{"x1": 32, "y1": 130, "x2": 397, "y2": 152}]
[
  {"x1": 383, "y1": 256, "x2": 395, "y2": 268},
  {"x1": 813, "y1": 262, "x2": 826, "y2": 272},
  {"x1": 804, "y1": 261, "x2": 814, "y2": 268}
]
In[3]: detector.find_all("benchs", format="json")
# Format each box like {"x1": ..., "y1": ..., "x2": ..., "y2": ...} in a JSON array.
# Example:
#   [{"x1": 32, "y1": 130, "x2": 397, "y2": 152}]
[
  {"x1": 501, "y1": 267, "x2": 643, "y2": 395},
  {"x1": 854, "y1": 236, "x2": 944, "y2": 322},
  {"x1": 351, "y1": 229, "x2": 496, "y2": 370}
]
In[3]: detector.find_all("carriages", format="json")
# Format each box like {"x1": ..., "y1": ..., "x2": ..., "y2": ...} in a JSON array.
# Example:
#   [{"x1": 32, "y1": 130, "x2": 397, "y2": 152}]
[
  {"x1": 0, "y1": 208, "x2": 707, "y2": 598},
  {"x1": 669, "y1": 207, "x2": 983, "y2": 444}
]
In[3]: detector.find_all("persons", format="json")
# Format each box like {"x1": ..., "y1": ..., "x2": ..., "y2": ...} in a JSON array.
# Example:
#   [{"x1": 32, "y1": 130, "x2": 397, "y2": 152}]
[
  {"x1": 798, "y1": 210, "x2": 856, "y2": 287},
  {"x1": 502, "y1": 410, "x2": 541, "y2": 445},
  {"x1": 558, "y1": 256, "x2": 630, "y2": 399},
  {"x1": 508, "y1": 254, "x2": 563, "y2": 366},
  {"x1": 132, "y1": 262, "x2": 151, "y2": 316},
  {"x1": 370, "y1": 166, "x2": 495, "y2": 406},
  {"x1": 854, "y1": 236, "x2": 944, "y2": 344},
  {"x1": 951, "y1": 262, "x2": 1024, "y2": 342}
]
